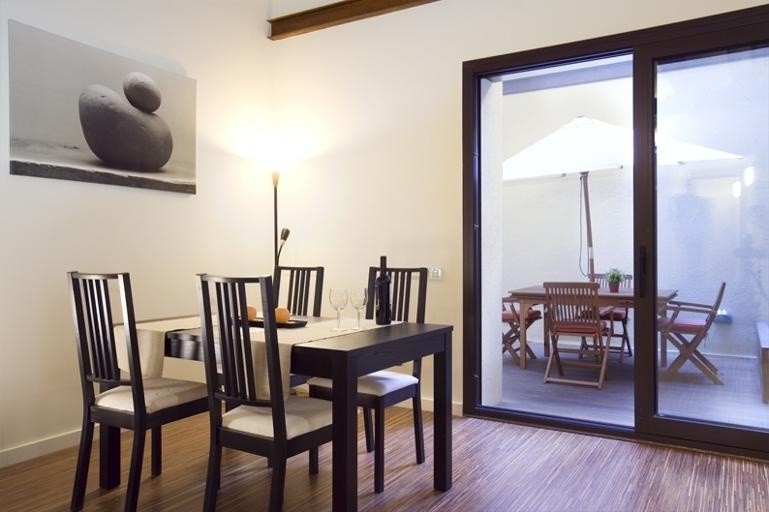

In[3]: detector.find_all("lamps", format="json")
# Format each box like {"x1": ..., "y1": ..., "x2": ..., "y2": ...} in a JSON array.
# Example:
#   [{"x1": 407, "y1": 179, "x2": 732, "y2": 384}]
[{"x1": 255, "y1": 128, "x2": 293, "y2": 266}]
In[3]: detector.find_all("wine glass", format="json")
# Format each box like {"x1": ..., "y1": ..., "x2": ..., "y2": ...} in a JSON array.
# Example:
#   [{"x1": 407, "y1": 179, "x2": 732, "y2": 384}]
[
  {"x1": 349, "y1": 285, "x2": 368, "y2": 331},
  {"x1": 329, "y1": 286, "x2": 349, "y2": 333}
]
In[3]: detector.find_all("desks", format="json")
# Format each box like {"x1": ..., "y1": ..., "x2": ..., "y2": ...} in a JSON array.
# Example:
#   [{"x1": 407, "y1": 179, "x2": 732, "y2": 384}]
[{"x1": 109, "y1": 312, "x2": 453, "y2": 512}]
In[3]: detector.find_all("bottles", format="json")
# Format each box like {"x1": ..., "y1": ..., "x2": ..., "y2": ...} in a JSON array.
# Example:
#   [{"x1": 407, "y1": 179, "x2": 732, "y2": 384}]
[{"x1": 374, "y1": 255, "x2": 393, "y2": 325}]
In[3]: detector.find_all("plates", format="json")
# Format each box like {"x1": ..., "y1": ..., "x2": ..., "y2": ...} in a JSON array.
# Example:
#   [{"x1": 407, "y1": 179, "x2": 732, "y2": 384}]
[{"x1": 238, "y1": 316, "x2": 308, "y2": 328}]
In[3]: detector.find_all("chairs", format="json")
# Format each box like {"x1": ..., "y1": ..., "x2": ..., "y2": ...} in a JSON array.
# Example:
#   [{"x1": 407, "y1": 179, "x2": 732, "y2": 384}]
[
  {"x1": 502, "y1": 274, "x2": 726, "y2": 390},
  {"x1": 268, "y1": 265, "x2": 325, "y2": 389},
  {"x1": 195, "y1": 272, "x2": 334, "y2": 512},
  {"x1": 68, "y1": 270, "x2": 226, "y2": 511},
  {"x1": 306, "y1": 267, "x2": 428, "y2": 495}
]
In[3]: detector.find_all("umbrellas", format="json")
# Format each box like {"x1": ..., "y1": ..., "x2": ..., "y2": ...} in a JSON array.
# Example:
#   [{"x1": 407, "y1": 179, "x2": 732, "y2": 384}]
[{"x1": 501, "y1": 115, "x2": 745, "y2": 363}]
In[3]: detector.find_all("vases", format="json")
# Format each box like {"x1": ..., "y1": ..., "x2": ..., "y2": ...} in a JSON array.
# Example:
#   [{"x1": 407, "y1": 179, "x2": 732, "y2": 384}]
[{"x1": 608, "y1": 269, "x2": 622, "y2": 292}]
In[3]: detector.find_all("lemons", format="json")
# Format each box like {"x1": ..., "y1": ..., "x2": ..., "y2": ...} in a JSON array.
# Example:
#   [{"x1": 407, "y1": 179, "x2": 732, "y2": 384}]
[
  {"x1": 246, "y1": 306, "x2": 258, "y2": 321},
  {"x1": 275, "y1": 307, "x2": 290, "y2": 323}
]
